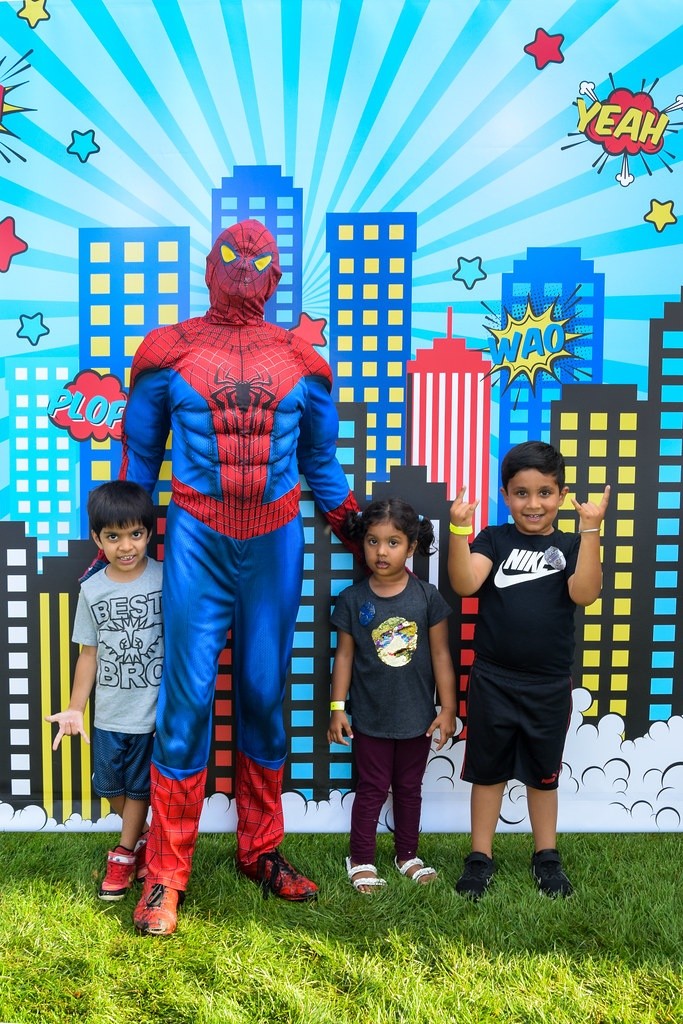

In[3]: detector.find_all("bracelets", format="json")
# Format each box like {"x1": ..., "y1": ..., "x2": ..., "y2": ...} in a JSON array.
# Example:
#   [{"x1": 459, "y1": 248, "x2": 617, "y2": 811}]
[
  {"x1": 449, "y1": 522, "x2": 474, "y2": 535},
  {"x1": 577, "y1": 529, "x2": 601, "y2": 534},
  {"x1": 329, "y1": 701, "x2": 345, "y2": 711}
]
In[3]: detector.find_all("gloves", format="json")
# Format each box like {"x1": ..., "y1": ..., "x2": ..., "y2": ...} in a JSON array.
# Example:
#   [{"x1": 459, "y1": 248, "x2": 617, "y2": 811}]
[{"x1": 76, "y1": 560, "x2": 104, "y2": 584}]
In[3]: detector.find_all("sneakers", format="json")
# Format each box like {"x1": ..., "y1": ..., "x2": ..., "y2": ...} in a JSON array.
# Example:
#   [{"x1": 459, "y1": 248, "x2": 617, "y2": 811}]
[
  {"x1": 132, "y1": 880, "x2": 178, "y2": 936},
  {"x1": 97, "y1": 829, "x2": 150, "y2": 901},
  {"x1": 530, "y1": 849, "x2": 574, "y2": 901},
  {"x1": 235, "y1": 849, "x2": 319, "y2": 902},
  {"x1": 457, "y1": 852, "x2": 496, "y2": 898}
]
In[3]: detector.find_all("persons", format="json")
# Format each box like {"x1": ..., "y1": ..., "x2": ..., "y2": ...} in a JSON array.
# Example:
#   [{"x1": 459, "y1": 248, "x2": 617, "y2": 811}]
[
  {"x1": 77, "y1": 217, "x2": 362, "y2": 936},
  {"x1": 447, "y1": 443, "x2": 611, "y2": 901},
  {"x1": 327, "y1": 497, "x2": 459, "y2": 894},
  {"x1": 45, "y1": 482, "x2": 162, "y2": 901}
]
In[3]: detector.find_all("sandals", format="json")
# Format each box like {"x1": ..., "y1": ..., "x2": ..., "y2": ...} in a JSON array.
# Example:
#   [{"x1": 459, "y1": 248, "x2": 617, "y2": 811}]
[
  {"x1": 395, "y1": 854, "x2": 436, "y2": 886},
  {"x1": 346, "y1": 856, "x2": 386, "y2": 896}
]
